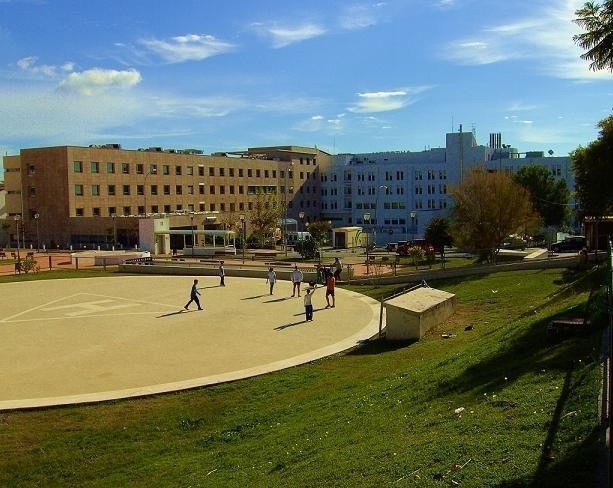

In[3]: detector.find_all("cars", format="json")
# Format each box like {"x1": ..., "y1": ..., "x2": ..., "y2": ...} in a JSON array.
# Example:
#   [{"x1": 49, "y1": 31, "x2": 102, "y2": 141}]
[
  {"x1": 500, "y1": 238, "x2": 528, "y2": 251},
  {"x1": 549, "y1": 234, "x2": 590, "y2": 254}
]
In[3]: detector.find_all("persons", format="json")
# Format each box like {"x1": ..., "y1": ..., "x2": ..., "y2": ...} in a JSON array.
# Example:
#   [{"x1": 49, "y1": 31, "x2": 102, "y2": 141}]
[
  {"x1": 290, "y1": 266, "x2": 303, "y2": 297},
  {"x1": 303, "y1": 285, "x2": 317, "y2": 322},
  {"x1": 316, "y1": 256, "x2": 342, "y2": 285},
  {"x1": 265, "y1": 267, "x2": 276, "y2": 295},
  {"x1": 323, "y1": 271, "x2": 336, "y2": 309},
  {"x1": 218, "y1": 262, "x2": 225, "y2": 286},
  {"x1": 183, "y1": 279, "x2": 203, "y2": 310}
]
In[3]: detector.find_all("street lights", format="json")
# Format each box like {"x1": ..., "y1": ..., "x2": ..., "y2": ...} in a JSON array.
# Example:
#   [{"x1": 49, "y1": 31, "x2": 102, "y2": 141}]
[
  {"x1": 144, "y1": 167, "x2": 157, "y2": 220},
  {"x1": 299, "y1": 212, "x2": 305, "y2": 263},
  {"x1": 33, "y1": 210, "x2": 43, "y2": 253},
  {"x1": 363, "y1": 212, "x2": 371, "y2": 274},
  {"x1": 13, "y1": 214, "x2": 21, "y2": 263},
  {"x1": 409, "y1": 210, "x2": 417, "y2": 271},
  {"x1": 238, "y1": 213, "x2": 245, "y2": 266},
  {"x1": 188, "y1": 211, "x2": 195, "y2": 262},
  {"x1": 111, "y1": 213, "x2": 118, "y2": 251}
]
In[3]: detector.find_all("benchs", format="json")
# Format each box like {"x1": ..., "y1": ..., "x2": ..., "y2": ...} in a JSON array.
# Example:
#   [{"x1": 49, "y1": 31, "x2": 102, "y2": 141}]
[
  {"x1": 213, "y1": 251, "x2": 235, "y2": 261},
  {"x1": 250, "y1": 252, "x2": 276, "y2": 262}
]
